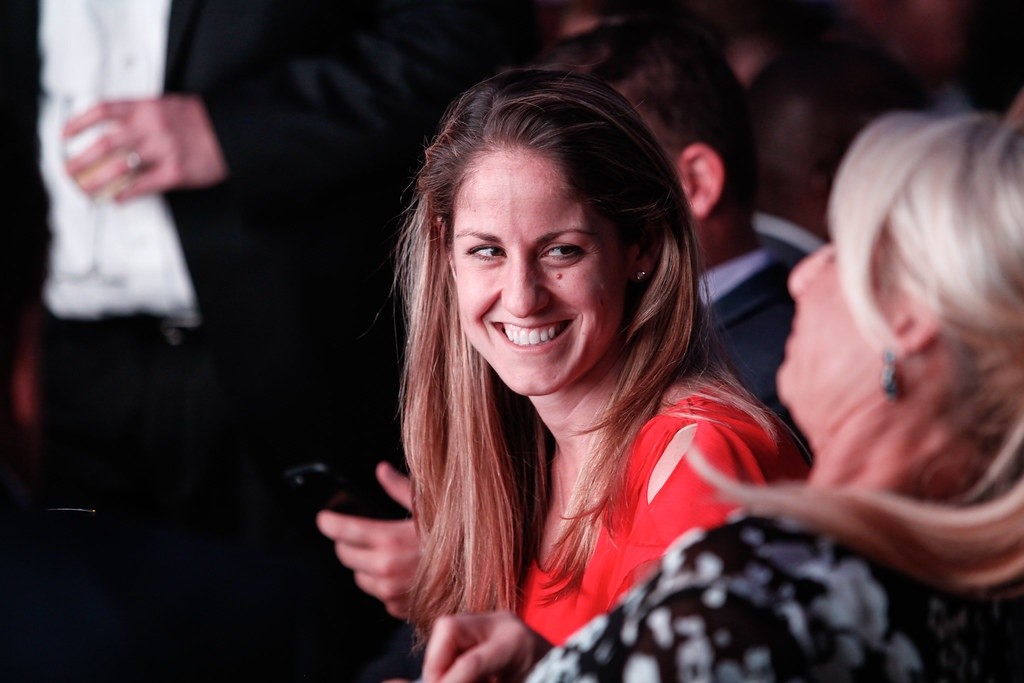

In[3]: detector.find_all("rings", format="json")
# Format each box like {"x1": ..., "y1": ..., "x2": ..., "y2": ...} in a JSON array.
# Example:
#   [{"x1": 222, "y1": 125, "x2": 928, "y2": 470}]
[{"x1": 128, "y1": 149, "x2": 145, "y2": 175}]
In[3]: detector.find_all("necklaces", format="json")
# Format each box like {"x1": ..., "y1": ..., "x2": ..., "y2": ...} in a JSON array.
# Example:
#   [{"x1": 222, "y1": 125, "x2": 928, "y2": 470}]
[{"x1": 552, "y1": 441, "x2": 588, "y2": 535}]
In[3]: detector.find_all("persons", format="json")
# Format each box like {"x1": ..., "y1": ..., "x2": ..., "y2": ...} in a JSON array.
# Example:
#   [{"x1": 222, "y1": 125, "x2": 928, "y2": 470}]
[
  {"x1": 551, "y1": 0, "x2": 1024, "y2": 238},
  {"x1": 0, "y1": 1, "x2": 516, "y2": 683},
  {"x1": 745, "y1": 42, "x2": 932, "y2": 246},
  {"x1": 313, "y1": 66, "x2": 808, "y2": 683},
  {"x1": 540, "y1": 9, "x2": 793, "y2": 420},
  {"x1": 521, "y1": 109, "x2": 1024, "y2": 683}
]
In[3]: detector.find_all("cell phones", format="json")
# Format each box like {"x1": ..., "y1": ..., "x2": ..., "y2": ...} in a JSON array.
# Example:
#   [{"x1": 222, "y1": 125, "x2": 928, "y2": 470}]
[{"x1": 285, "y1": 463, "x2": 410, "y2": 522}]
